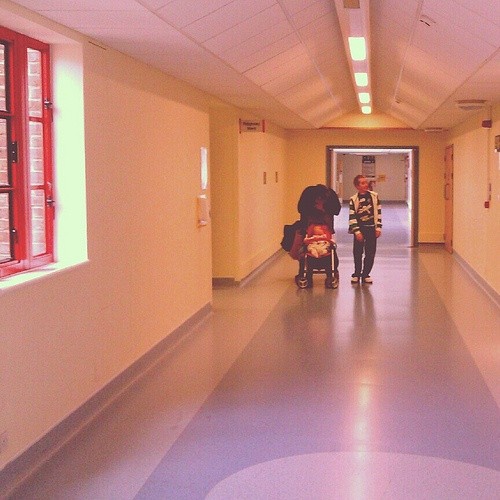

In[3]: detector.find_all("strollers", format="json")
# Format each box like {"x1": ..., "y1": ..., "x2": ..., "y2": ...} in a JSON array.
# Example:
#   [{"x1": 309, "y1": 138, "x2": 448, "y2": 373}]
[{"x1": 295, "y1": 220, "x2": 340, "y2": 289}]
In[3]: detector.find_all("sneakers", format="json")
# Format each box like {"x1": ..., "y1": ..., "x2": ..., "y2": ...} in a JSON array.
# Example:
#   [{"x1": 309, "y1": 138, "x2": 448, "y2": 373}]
[
  {"x1": 350, "y1": 276, "x2": 360, "y2": 282},
  {"x1": 362, "y1": 276, "x2": 373, "y2": 283}
]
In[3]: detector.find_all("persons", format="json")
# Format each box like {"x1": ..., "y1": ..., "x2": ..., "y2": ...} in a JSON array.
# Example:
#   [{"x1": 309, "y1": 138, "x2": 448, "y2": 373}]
[
  {"x1": 307, "y1": 224, "x2": 331, "y2": 258},
  {"x1": 346, "y1": 173, "x2": 383, "y2": 284},
  {"x1": 296, "y1": 183, "x2": 343, "y2": 290}
]
